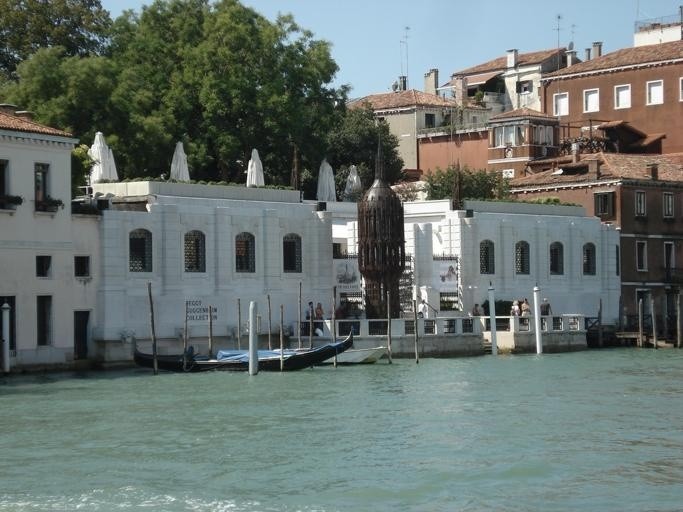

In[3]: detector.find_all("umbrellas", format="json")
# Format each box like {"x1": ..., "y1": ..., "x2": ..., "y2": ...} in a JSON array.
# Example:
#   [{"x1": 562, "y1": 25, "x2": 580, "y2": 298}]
[
  {"x1": 340, "y1": 163, "x2": 362, "y2": 202},
  {"x1": 87, "y1": 131, "x2": 119, "y2": 183},
  {"x1": 313, "y1": 158, "x2": 337, "y2": 201},
  {"x1": 244, "y1": 148, "x2": 265, "y2": 188},
  {"x1": 169, "y1": 139, "x2": 190, "y2": 185}
]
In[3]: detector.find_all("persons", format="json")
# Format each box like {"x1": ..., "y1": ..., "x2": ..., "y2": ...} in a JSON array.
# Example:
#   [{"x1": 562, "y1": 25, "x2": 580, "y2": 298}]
[
  {"x1": 471, "y1": 303, "x2": 481, "y2": 316},
  {"x1": 416, "y1": 298, "x2": 427, "y2": 321},
  {"x1": 520, "y1": 297, "x2": 532, "y2": 326},
  {"x1": 303, "y1": 301, "x2": 315, "y2": 336},
  {"x1": 314, "y1": 303, "x2": 325, "y2": 336},
  {"x1": 539, "y1": 297, "x2": 553, "y2": 330},
  {"x1": 507, "y1": 300, "x2": 520, "y2": 331},
  {"x1": 334, "y1": 302, "x2": 346, "y2": 319}
]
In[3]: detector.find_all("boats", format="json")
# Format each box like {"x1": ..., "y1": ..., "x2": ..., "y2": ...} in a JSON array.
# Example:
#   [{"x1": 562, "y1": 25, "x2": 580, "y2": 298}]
[
  {"x1": 274, "y1": 346, "x2": 389, "y2": 365},
  {"x1": 133, "y1": 325, "x2": 353, "y2": 372}
]
